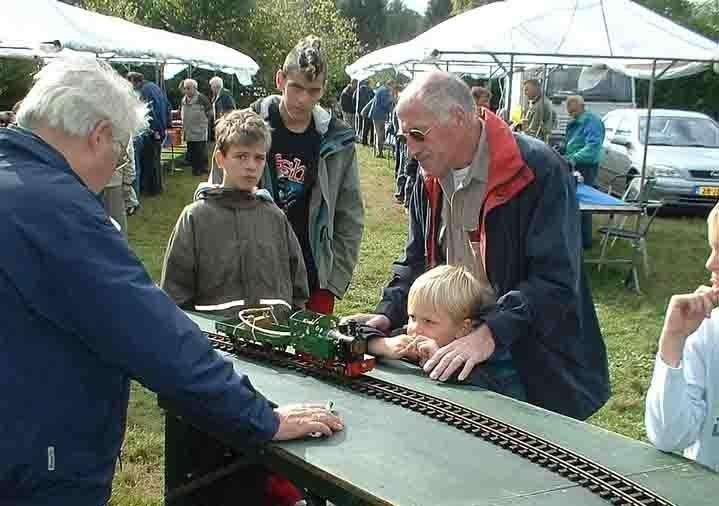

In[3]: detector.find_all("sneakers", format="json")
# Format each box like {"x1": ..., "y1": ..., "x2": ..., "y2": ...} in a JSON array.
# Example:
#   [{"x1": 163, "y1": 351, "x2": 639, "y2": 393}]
[{"x1": 127, "y1": 204, "x2": 140, "y2": 216}]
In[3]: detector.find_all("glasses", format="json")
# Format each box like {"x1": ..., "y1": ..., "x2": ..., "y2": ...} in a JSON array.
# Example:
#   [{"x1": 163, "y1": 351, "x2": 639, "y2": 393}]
[
  {"x1": 112, "y1": 129, "x2": 132, "y2": 171},
  {"x1": 396, "y1": 116, "x2": 450, "y2": 144}
]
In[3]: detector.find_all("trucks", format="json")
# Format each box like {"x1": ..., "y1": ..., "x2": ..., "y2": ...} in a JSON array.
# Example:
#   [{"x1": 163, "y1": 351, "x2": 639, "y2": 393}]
[{"x1": 499, "y1": 68, "x2": 638, "y2": 147}]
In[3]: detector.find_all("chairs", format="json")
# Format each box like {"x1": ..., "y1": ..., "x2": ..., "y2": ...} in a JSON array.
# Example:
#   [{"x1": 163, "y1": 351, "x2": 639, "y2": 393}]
[
  {"x1": 593, "y1": 173, "x2": 657, "y2": 253},
  {"x1": 595, "y1": 197, "x2": 667, "y2": 282}
]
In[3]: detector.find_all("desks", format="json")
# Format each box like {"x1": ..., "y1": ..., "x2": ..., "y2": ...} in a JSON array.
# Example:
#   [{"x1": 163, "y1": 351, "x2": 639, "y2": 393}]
[{"x1": 576, "y1": 181, "x2": 643, "y2": 297}]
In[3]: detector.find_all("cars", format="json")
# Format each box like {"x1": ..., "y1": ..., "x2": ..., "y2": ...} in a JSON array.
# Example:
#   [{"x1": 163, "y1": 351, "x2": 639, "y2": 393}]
[{"x1": 590, "y1": 105, "x2": 719, "y2": 212}]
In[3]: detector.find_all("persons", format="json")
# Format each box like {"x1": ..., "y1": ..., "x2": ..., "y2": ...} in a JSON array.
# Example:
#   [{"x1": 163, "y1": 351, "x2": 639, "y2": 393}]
[
  {"x1": 1, "y1": 34, "x2": 365, "y2": 506},
  {"x1": 342, "y1": 70, "x2": 611, "y2": 421},
  {"x1": 645, "y1": 198, "x2": 719, "y2": 476}
]
